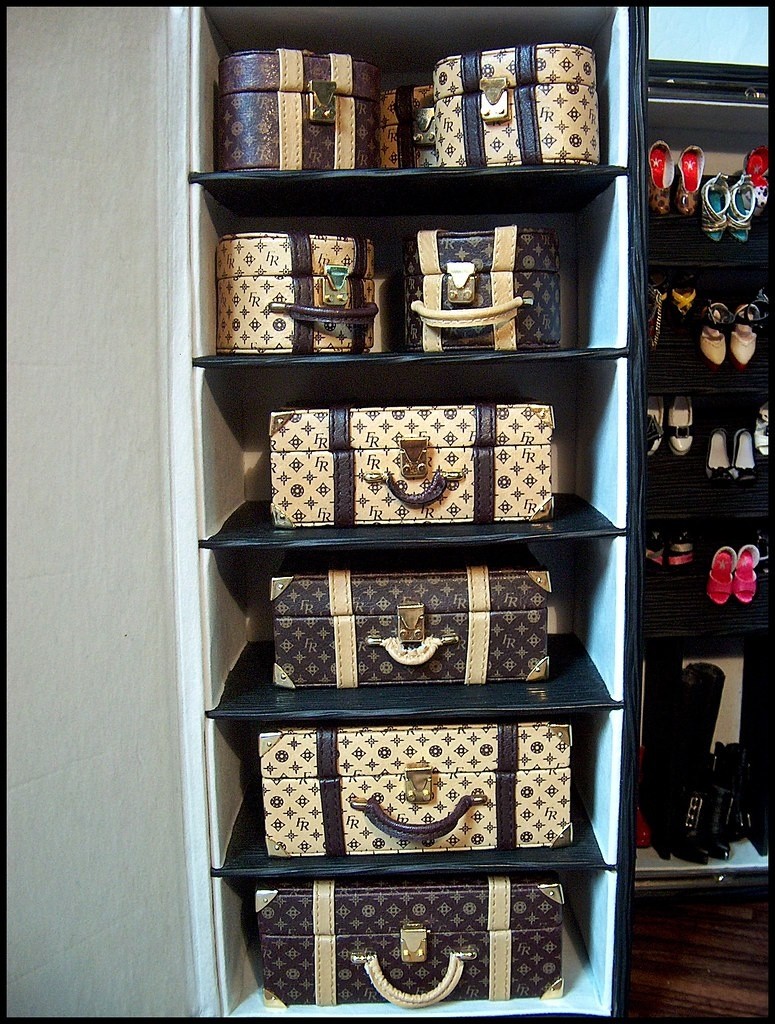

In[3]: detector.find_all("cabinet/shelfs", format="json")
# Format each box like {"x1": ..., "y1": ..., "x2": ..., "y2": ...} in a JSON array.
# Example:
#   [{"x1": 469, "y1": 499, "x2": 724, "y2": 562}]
[
  {"x1": 620, "y1": 96, "x2": 775, "y2": 891},
  {"x1": 185, "y1": 4, "x2": 648, "y2": 1021}
]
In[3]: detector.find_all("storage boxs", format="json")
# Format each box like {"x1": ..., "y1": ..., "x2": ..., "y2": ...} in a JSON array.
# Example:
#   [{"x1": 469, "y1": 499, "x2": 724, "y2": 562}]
[
  {"x1": 402, "y1": 226, "x2": 563, "y2": 354},
  {"x1": 255, "y1": 871, "x2": 566, "y2": 1007},
  {"x1": 256, "y1": 720, "x2": 576, "y2": 860},
  {"x1": 268, "y1": 543, "x2": 553, "y2": 688},
  {"x1": 214, "y1": 229, "x2": 379, "y2": 356},
  {"x1": 266, "y1": 401, "x2": 558, "y2": 528},
  {"x1": 434, "y1": 43, "x2": 601, "y2": 169},
  {"x1": 372, "y1": 86, "x2": 441, "y2": 169},
  {"x1": 217, "y1": 47, "x2": 384, "y2": 172}
]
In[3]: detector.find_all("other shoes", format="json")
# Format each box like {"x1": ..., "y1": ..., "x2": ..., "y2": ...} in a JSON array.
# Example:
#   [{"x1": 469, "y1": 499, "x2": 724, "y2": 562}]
[{"x1": 647, "y1": 266, "x2": 696, "y2": 323}]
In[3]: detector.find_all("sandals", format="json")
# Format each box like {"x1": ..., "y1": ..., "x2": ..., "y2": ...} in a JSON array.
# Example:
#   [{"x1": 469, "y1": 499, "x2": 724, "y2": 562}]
[
  {"x1": 644, "y1": 524, "x2": 693, "y2": 578},
  {"x1": 705, "y1": 428, "x2": 758, "y2": 489},
  {"x1": 757, "y1": 531, "x2": 769, "y2": 574},
  {"x1": 754, "y1": 403, "x2": 769, "y2": 455},
  {"x1": 701, "y1": 173, "x2": 757, "y2": 243},
  {"x1": 706, "y1": 544, "x2": 759, "y2": 605}
]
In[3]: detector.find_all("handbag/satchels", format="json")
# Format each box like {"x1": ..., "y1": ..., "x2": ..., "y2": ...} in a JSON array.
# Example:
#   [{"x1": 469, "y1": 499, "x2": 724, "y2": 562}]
[
  {"x1": 213, "y1": 44, "x2": 599, "y2": 172},
  {"x1": 216, "y1": 232, "x2": 388, "y2": 354},
  {"x1": 406, "y1": 225, "x2": 563, "y2": 350}
]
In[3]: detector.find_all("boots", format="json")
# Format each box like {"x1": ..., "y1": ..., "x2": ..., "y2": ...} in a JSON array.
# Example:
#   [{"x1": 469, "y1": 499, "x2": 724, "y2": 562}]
[{"x1": 636, "y1": 644, "x2": 752, "y2": 864}]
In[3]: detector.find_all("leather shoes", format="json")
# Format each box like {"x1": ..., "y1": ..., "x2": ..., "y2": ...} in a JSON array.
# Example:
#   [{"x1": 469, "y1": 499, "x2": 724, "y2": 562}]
[
  {"x1": 743, "y1": 144, "x2": 768, "y2": 216},
  {"x1": 645, "y1": 396, "x2": 694, "y2": 456},
  {"x1": 646, "y1": 139, "x2": 705, "y2": 216},
  {"x1": 700, "y1": 287, "x2": 771, "y2": 373}
]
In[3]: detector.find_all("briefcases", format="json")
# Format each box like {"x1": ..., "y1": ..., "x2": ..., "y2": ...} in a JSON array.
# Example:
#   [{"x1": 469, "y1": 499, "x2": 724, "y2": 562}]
[
  {"x1": 255, "y1": 869, "x2": 567, "y2": 1008},
  {"x1": 259, "y1": 722, "x2": 573, "y2": 856},
  {"x1": 267, "y1": 393, "x2": 555, "y2": 524},
  {"x1": 270, "y1": 542, "x2": 553, "y2": 688}
]
